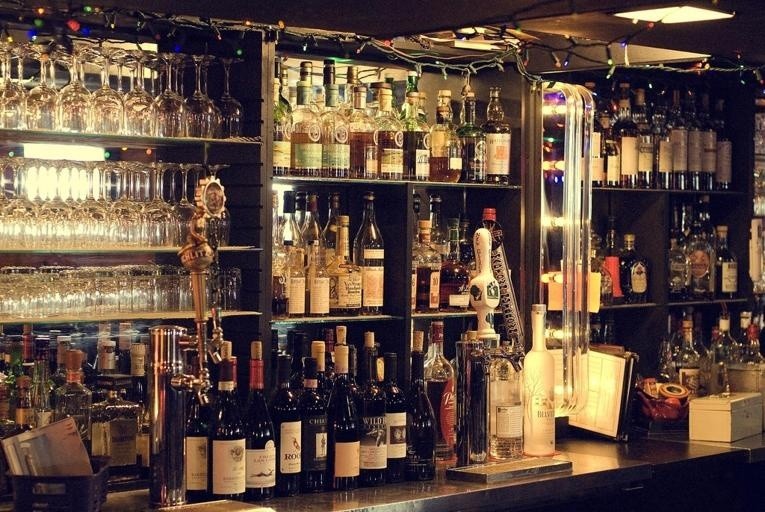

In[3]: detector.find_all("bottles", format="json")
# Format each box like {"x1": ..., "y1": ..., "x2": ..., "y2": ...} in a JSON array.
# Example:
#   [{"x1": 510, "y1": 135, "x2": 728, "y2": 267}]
[
  {"x1": 294, "y1": 187, "x2": 306, "y2": 226},
  {"x1": 604, "y1": 228, "x2": 625, "y2": 305},
  {"x1": 652, "y1": 86, "x2": 674, "y2": 190},
  {"x1": 423, "y1": 194, "x2": 444, "y2": 243},
  {"x1": 714, "y1": 225, "x2": 738, "y2": 300},
  {"x1": 290, "y1": 80, "x2": 321, "y2": 177},
  {"x1": 633, "y1": 88, "x2": 656, "y2": 189},
  {"x1": 424, "y1": 322, "x2": 458, "y2": 470},
  {"x1": 293, "y1": 61, "x2": 313, "y2": 109},
  {"x1": 686, "y1": 199, "x2": 715, "y2": 300},
  {"x1": 621, "y1": 233, "x2": 650, "y2": 304},
  {"x1": 402, "y1": 90, "x2": 430, "y2": 180},
  {"x1": 709, "y1": 312, "x2": 737, "y2": 394},
  {"x1": 211, "y1": 339, "x2": 245, "y2": 501},
  {"x1": 301, "y1": 357, "x2": 330, "y2": 493},
  {"x1": 590, "y1": 119, "x2": 606, "y2": 187},
  {"x1": 1, "y1": 321, "x2": 152, "y2": 464},
  {"x1": 715, "y1": 98, "x2": 733, "y2": 191},
  {"x1": 318, "y1": 59, "x2": 334, "y2": 109},
  {"x1": 487, "y1": 340, "x2": 525, "y2": 461},
  {"x1": 585, "y1": 81, "x2": 600, "y2": 132},
  {"x1": 478, "y1": 87, "x2": 514, "y2": 184},
  {"x1": 302, "y1": 190, "x2": 324, "y2": 246},
  {"x1": 342, "y1": 67, "x2": 358, "y2": 107},
  {"x1": 666, "y1": 229, "x2": 691, "y2": 301},
  {"x1": 430, "y1": 90, "x2": 462, "y2": 182},
  {"x1": 700, "y1": 93, "x2": 718, "y2": 190},
  {"x1": 523, "y1": 304, "x2": 557, "y2": 456},
  {"x1": 374, "y1": 83, "x2": 402, "y2": 180},
  {"x1": 743, "y1": 323, "x2": 762, "y2": 364},
  {"x1": 328, "y1": 216, "x2": 362, "y2": 315},
  {"x1": 459, "y1": 91, "x2": 486, "y2": 183},
  {"x1": 243, "y1": 339, "x2": 277, "y2": 502},
  {"x1": 655, "y1": 316, "x2": 678, "y2": 381},
  {"x1": 412, "y1": 219, "x2": 442, "y2": 311},
  {"x1": 380, "y1": 350, "x2": 407, "y2": 480},
  {"x1": 588, "y1": 233, "x2": 613, "y2": 308},
  {"x1": 273, "y1": 352, "x2": 304, "y2": 496},
  {"x1": 347, "y1": 88, "x2": 378, "y2": 178},
  {"x1": 357, "y1": 331, "x2": 389, "y2": 485},
  {"x1": 322, "y1": 83, "x2": 349, "y2": 178},
  {"x1": 328, "y1": 346, "x2": 362, "y2": 491},
  {"x1": 311, "y1": 340, "x2": 326, "y2": 407},
  {"x1": 271, "y1": 192, "x2": 288, "y2": 317},
  {"x1": 272, "y1": 56, "x2": 293, "y2": 175},
  {"x1": 454, "y1": 330, "x2": 487, "y2": 468},
  {"x1": 309, "y1": 240, "x2": 330, "y2": 315},
  {"x1": 413, "y1": 330, "x2": 437, "y2": 482},
  {"x1": 283, "y1": 191, "x2": 304, "y2": 318},
  {"x1": 603, "y1": 125, "x2": 620, "y2": 187},
  {"x1": 596, "y1": 94, "x2": 610, "y2": 128},
  {"x1": 184, "y1": 390, "x2": 211, "y2": 499},
  {"x1": 614, "y1": 84, "x2": 637, "y2": 188},
  {"x1": 668, "y1": 88, "x2": 688, "y2": 191},
  {"x1": 286, "y1": 249, "x2": 306, "y2": 318},
  {"x1": 687, "y1": 89, "x2": 704, "y2": 191},
  {"x1": 320, "y1": 191, "x2": 341, "y2": 274},
  {"x1": 677, "y1": 317, "x2": 699, "y2": 397},
  {"x1": 438, "y1": 217, "x2": 471, "y2": 311}
]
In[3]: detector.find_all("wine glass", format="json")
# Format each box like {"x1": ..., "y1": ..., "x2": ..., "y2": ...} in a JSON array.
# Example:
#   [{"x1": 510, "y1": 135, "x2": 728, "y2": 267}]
[
  {"x1": 0, "y1": 157, "x2": 230, "y2": 245},
  {"x1": 1, "y1": 36, "x2": 244, "y2": 139},
  {"x1": 1, "y1": 264, "x2": 239, "y2": 314}
]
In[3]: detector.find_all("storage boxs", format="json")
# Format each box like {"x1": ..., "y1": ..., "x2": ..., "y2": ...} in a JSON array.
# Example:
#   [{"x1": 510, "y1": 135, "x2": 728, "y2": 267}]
[{"x1": 689, "y1": 389, "x2": 762, "y2": 444}]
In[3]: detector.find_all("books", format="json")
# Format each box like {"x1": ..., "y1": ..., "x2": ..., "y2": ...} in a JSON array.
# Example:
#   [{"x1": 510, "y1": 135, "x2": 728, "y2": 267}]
[
  {"x1": 0, "y1": 417, "x2": 94, "y2": 476},
  {"x1": 568, "y1": 349, "x2": 639, "y2": 442}
]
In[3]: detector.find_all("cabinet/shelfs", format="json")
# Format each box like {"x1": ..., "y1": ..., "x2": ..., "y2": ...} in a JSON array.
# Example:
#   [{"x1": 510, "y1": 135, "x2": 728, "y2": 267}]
[
  {"x1": 526, "y1": 59, "x2": 747, "y2": 417},
  {"x1": 0, "y1": 22, "x2": 267, "y2": 327},
  {"x1": 268, "y1": 26, "x2": 527, "y2": 376}
]
